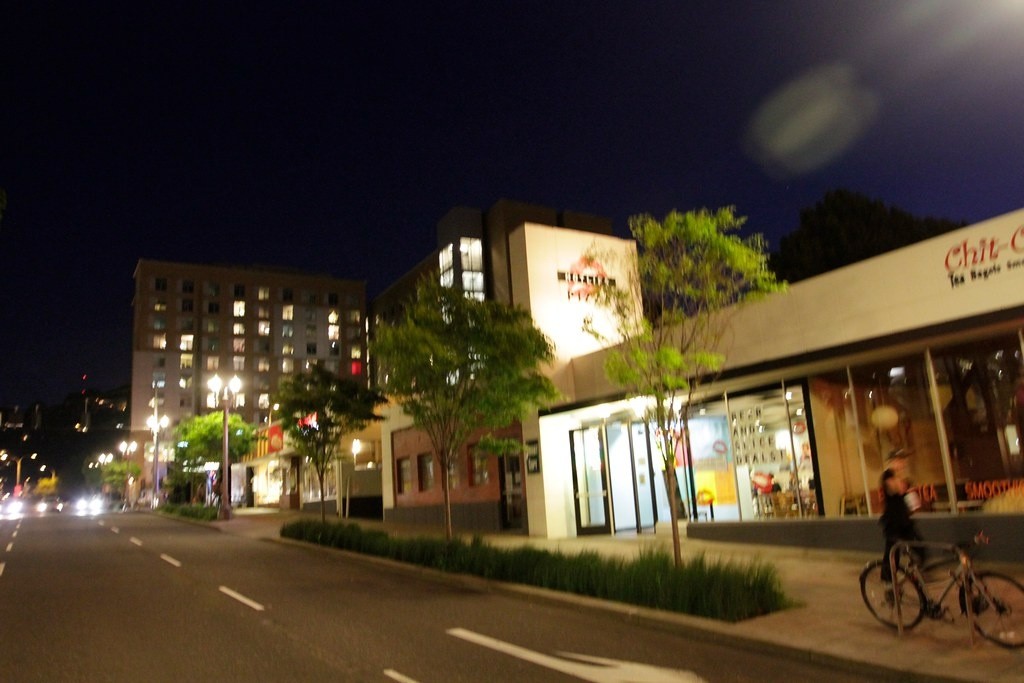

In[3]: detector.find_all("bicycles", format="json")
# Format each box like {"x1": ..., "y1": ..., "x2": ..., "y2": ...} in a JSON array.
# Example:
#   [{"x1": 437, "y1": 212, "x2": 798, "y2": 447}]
[{"x1": 857, "y1": 531, "x2": 1024, "y2": 652}]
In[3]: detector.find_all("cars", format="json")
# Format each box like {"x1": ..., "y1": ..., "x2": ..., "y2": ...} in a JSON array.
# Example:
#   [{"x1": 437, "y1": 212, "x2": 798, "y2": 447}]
[{"x1": 40, "y1": 496, "x2": 62, "y2": 511}]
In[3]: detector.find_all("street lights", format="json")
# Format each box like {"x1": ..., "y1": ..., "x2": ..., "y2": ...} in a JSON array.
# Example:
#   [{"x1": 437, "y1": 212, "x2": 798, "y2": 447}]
[
  {"x1": 119, "y1": 440, "x2": 137, "y2": 509},
  {"x1": 2, "y1": 443, "x2": 114, "y2": 499},
  {"x1": 145, "y1": 414, "x2": 171, "y2": 509},
  {"x1": 206, "y1": 372, "x2": 242, "y2": 521}
]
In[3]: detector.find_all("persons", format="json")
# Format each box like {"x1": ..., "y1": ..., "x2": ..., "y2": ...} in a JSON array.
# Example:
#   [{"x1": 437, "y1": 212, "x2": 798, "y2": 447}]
[{"x1": 879, "y1": 468, "x2": 929, "y2": 583}]
